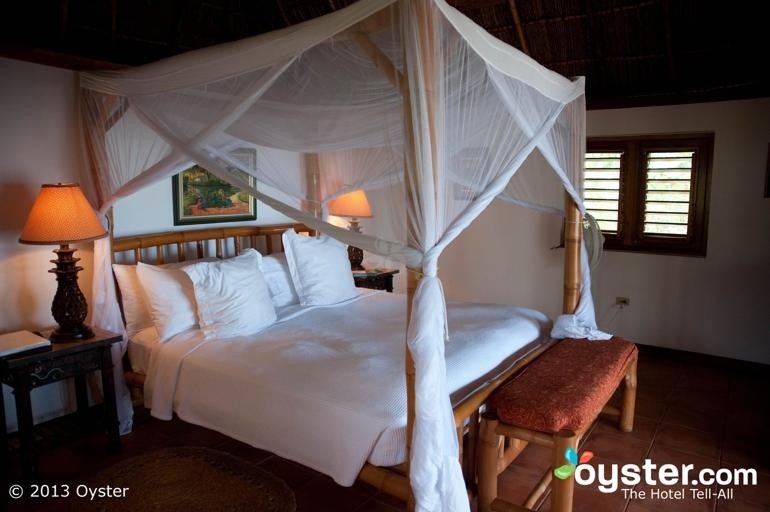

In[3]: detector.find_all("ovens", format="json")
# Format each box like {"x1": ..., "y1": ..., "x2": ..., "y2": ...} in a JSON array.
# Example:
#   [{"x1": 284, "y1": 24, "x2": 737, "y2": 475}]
[{"x1": 78, "y1": 0, "x2": 613, "y2": 512}]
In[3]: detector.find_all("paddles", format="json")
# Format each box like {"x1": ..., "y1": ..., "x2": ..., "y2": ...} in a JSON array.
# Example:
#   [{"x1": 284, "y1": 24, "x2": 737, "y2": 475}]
[{"x1": 71, "y1": 446, "x2": 296, "y2": 512}]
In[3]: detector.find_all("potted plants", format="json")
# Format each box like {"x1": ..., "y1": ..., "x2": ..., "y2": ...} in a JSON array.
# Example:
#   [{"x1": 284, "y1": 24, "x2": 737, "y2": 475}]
[{"x1": 172, "y1": 149, "x2": 256, "y2": 225}]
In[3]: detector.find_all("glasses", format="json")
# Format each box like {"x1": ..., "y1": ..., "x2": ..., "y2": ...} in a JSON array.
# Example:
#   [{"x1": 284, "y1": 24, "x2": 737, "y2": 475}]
[
  {"x1": 18, "y1": 182, "x2": 107, "y2": 343},
  {"x1": 327, "y1": 183, "x2": 373, "y2": 270}
]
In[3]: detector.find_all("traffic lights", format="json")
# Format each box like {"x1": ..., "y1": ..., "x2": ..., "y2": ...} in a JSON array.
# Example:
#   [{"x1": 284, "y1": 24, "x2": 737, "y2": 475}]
[
  {"x1": 113, "y1": 264, "x2": 152, "y2": 340},
  {"x1": 136, "y1": 257, "x2": 220, "y2": 343},
  {"x1": 180, "y1": 248, "x2": 277, "y2": 339},
  {"x1": 282, "y1": 227, "x2": 359, "y2": 305},
  {"x1": 262, "y1": 251, "x2": 299, "y2": 308}
]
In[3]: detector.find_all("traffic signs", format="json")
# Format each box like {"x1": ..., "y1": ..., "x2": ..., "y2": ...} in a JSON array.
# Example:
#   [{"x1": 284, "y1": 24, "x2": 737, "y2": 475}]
[{"x1": 616, "y1": 296, "x2": 629, "y2": 305}]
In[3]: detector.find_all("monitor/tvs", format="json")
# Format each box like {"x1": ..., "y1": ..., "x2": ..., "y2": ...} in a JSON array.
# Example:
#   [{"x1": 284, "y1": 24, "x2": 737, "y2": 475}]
[{"x1": 0, "y1": 330, "x2": 51, "y2": 359}]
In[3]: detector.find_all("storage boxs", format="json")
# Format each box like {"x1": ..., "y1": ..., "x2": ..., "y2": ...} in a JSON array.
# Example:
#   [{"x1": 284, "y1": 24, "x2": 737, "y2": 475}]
[{"x1": 477, "y1": 337, "x2": 638, "y2": 512}]
